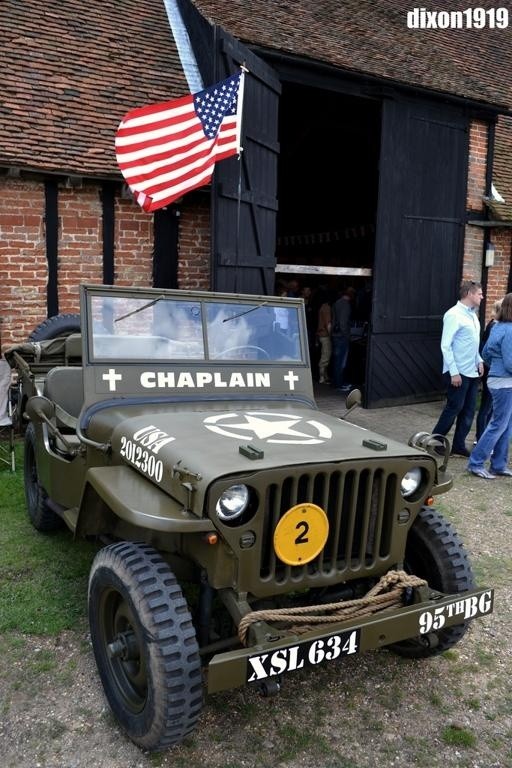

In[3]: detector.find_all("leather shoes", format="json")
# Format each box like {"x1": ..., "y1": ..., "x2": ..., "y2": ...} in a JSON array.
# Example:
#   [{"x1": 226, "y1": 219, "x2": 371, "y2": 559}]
[
  {"x1": 319, "y1": 376, "x2": 334, "y2": 385},
  {"x1": 489, "y1": 467, "x2": 512, "y2": 476},
  {"x1": 465, "y1": 465, "x2": 496, "y2": 479}
]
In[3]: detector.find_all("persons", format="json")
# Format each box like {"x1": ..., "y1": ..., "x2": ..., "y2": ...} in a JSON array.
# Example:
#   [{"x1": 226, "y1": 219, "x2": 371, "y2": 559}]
[
  {"x1": 476, "y1": 298, "x2": 504, "y2": 455},
  {"x1": 432, "y1": 281, "x2": 485, "y2": 458},
  {"x1": 280, "y1": 279, "x2": 355, "y2": 391},
  {"x1": 465, "y1": 293, "x2": 512, "y2": 479}
]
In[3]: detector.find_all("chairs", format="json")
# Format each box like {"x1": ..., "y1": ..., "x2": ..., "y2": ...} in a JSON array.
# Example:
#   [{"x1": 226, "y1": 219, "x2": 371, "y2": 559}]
[
  {"x1": 0, "y1": 358, "x2": 19, "y2": 471},
  {"x1": 43, "y1": 366, "x2": 85, "y2": 447}
]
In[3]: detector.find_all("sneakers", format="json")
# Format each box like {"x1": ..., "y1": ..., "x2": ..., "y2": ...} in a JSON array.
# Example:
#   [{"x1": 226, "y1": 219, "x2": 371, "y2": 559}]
[
  {"x1": 336, "y1": 382, "x2": 352, "y2": 391},
  {"x1": 432, "y1": 444, "x2": 452, "y2": 458},
  {"x1": 450, "y1": 447, "x2": 471, "y2": 459}
]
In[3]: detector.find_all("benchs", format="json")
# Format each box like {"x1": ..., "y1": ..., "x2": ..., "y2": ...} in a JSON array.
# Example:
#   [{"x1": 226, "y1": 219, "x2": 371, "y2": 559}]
[{"x1": 62, "y1": 334, "x2": 172, "y2": 367}]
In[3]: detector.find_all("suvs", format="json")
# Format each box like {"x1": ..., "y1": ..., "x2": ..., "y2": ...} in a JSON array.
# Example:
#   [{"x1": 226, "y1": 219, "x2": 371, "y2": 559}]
[{"x1": 2, "y1": 282, "x2": 499, "y2": 753}]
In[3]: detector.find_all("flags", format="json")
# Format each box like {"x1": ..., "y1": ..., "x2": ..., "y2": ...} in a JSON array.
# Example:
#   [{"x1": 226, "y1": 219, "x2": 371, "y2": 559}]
[{"x1": 115, "y1": 70, "x2": 244, "y2": 212}]
